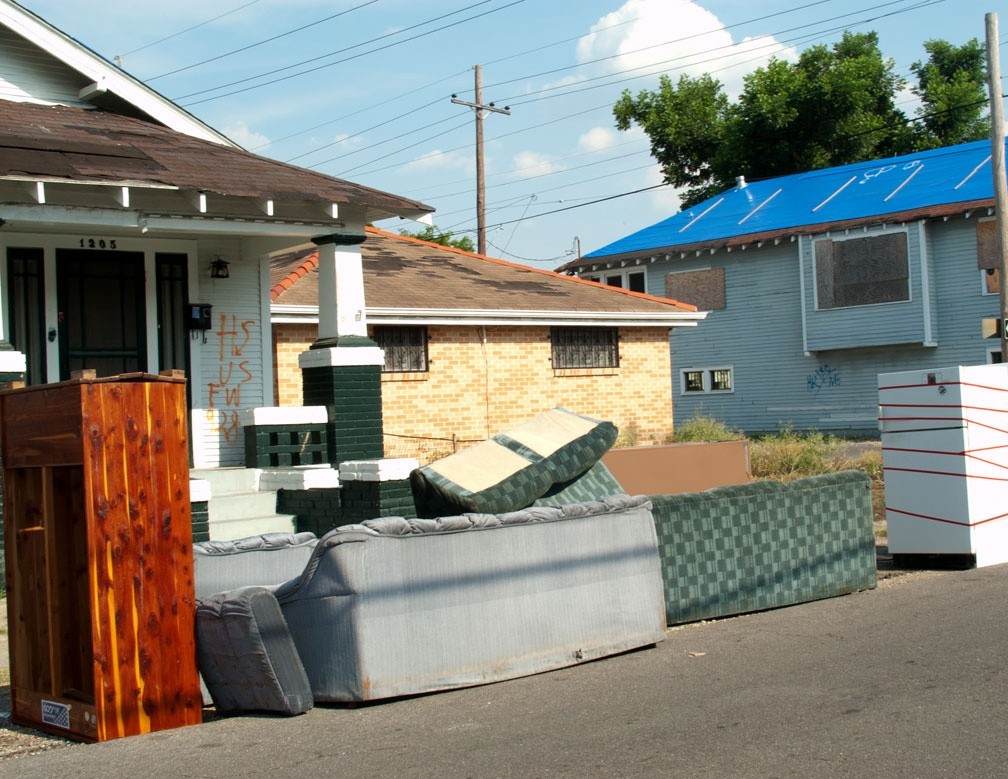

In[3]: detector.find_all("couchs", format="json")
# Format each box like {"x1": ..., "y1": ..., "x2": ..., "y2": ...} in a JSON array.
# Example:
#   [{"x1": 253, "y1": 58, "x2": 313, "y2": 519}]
[{"x1": 192, "y1": 407, "x2": 873, "y2": 717}]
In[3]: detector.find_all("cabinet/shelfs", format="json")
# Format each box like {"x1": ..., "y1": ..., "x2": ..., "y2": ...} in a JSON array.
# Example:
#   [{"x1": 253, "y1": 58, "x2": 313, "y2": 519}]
[
  {"x1": 878, "y1": 362, "x2": 1008, "y2": 568},
  {"x1": 0, "y1": 369, "x2": 203, "y2": 742}
]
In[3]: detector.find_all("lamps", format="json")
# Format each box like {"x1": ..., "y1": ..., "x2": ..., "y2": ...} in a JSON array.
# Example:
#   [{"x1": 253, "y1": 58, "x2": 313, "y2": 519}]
[{"x1": 210, "y1": 259, "x2": 230, "y2": 278}]
